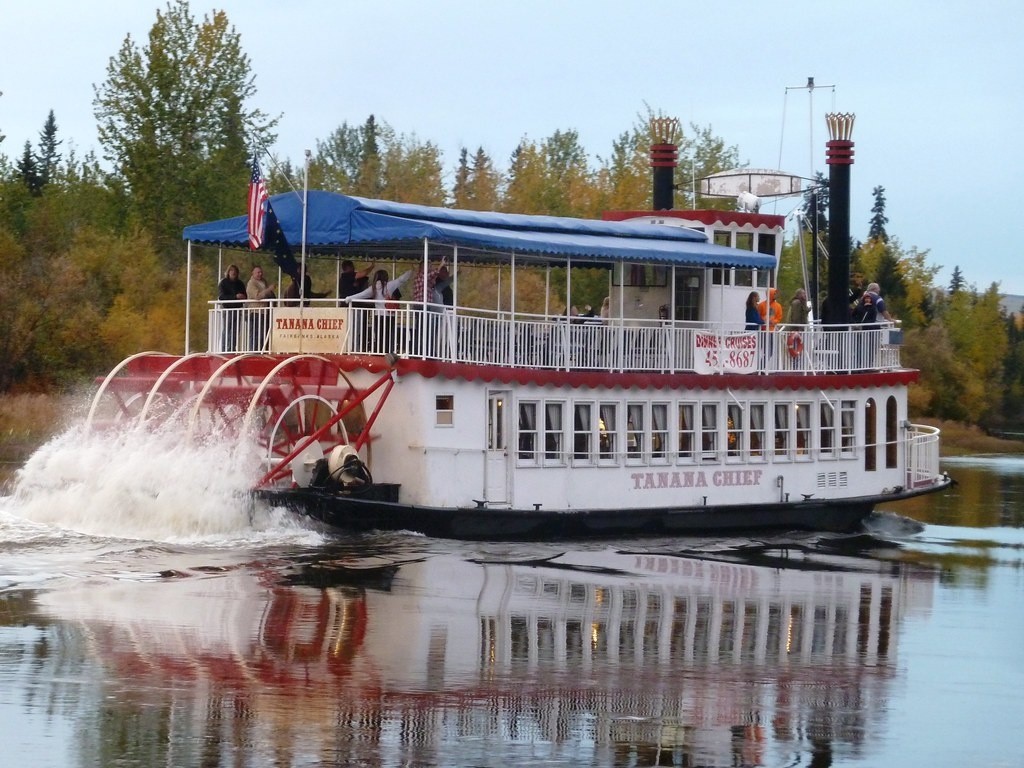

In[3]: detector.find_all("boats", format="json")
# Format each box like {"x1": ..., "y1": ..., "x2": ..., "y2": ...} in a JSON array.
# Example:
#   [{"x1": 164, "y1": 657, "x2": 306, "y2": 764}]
[{"x1": 88, "y1": 112, "x2": 959, "y2": 542}]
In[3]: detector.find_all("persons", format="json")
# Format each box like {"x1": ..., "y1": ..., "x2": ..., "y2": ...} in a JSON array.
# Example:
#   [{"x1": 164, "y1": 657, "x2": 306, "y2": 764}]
[
  {"x1": 217, "y1": 255, "x2": 463, "y2": 360},
  {"x1": 743, "y1": 284, "x2": 897, "y2": 374}
]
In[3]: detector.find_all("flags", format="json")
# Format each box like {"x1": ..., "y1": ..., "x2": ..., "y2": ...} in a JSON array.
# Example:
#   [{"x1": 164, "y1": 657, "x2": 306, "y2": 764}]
[{"x1": 247, "y1": 154, "x2": 269, "y2": 251}]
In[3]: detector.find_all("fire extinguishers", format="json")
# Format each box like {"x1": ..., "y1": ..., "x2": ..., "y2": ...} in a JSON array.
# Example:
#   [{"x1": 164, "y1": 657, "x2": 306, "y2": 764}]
[{"x1": 658, "y1": 304, "x2": 669, "y2": 327}]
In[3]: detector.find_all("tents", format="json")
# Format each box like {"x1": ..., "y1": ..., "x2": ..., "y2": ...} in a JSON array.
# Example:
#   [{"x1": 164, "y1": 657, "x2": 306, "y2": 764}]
[{"x1": 182, "y1": 187, "x2": 778, "y2": 376}]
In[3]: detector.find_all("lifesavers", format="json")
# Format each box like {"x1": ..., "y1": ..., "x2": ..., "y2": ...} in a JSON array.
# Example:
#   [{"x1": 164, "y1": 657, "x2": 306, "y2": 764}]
[{"x1": 787, "y1": 331, "x2": 803, "y2": 357}]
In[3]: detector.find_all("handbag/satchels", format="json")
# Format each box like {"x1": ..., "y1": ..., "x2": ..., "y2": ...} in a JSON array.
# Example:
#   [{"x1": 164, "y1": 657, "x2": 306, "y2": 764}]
[{"x1": 385, "y1": 297, "x2": 400, "y2": 314}]
